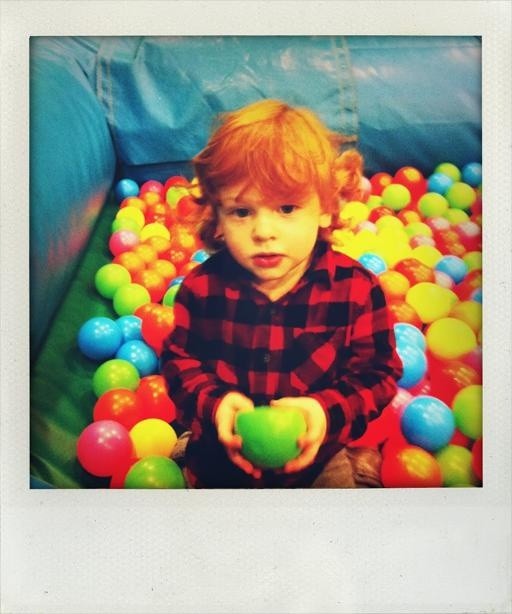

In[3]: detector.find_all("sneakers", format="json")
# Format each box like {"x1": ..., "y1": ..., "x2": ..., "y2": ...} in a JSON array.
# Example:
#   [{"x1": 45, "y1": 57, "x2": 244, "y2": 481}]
[
  {"x1": 169, "y1": 431, "x2": 193, "y2": 470},
  {"x1": 347, "y1": 446, "x2": 382, "y2": 488}
]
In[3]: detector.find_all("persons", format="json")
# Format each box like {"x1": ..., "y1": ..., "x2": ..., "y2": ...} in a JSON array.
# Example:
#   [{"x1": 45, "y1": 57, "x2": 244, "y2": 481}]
[{"x1": 160, "y1": 97, "x2": 404, "y2": 488}]
